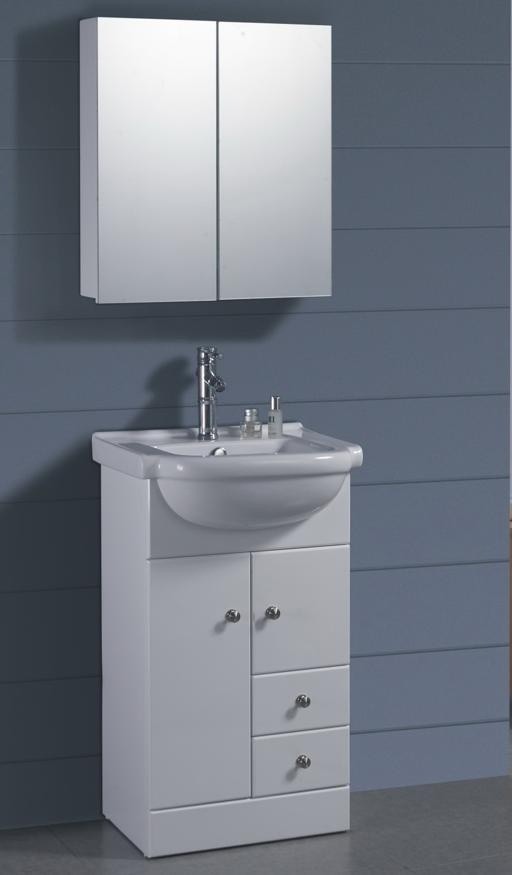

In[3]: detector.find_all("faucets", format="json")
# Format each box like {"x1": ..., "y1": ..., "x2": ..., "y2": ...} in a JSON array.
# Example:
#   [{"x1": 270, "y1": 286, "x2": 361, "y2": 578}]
[{"x1": 197, "y1": 344, "x2": 224, "y2": 439}]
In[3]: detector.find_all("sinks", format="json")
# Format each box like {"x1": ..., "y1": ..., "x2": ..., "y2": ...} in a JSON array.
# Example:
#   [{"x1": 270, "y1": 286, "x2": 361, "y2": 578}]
[{"x1": 93, "y1": 419, "x2": 363, "y2": 532}]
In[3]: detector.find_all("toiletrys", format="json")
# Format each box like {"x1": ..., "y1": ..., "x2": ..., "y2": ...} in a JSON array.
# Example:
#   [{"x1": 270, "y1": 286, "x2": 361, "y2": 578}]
[
  {"x1": 266, "y1": 395, "x2": 283, "y2": 439},
  {"x1": 238, "y1": 408, "x2": 263, "y2": 440}
]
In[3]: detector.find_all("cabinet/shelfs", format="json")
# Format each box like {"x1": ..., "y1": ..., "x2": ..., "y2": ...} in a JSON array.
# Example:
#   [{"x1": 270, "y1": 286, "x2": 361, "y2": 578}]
[
  {"x1": 79, "y1": 17, "x2": 334, "y2": 303},
  {"x1": 101, "y1": 470, "x2": 350, "y2": 860}
]
